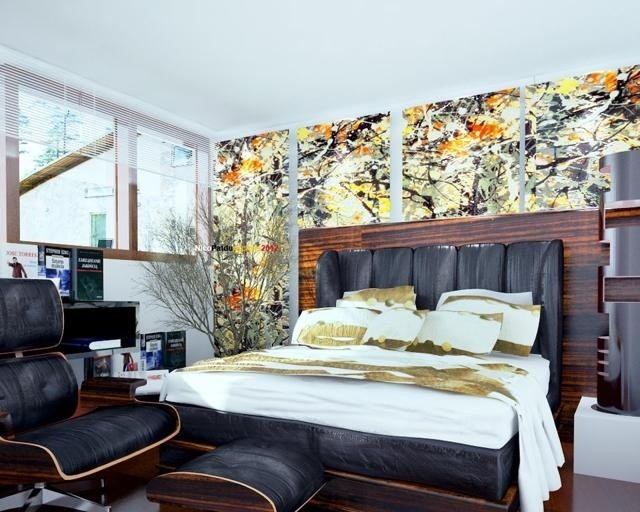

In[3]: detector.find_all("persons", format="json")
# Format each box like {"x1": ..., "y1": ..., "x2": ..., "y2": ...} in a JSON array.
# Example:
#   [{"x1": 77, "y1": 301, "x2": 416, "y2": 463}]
[
  {"x1": 121, "y1": 351, "x2": 135, "y2": 372},
  {"x1": 7, "y1": 257, "x2": 27, "y2": 278}
]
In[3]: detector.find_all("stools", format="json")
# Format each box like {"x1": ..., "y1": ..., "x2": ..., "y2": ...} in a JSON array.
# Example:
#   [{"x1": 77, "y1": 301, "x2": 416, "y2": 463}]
[{"x1": 146, "y1": 441, "x2": 331, "y2": 511}]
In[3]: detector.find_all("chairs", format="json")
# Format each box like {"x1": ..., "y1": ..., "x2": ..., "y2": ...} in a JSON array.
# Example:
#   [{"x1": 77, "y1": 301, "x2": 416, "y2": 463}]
[{"x1": 0, "y1": 278, "x2": 179, "y2": 504}]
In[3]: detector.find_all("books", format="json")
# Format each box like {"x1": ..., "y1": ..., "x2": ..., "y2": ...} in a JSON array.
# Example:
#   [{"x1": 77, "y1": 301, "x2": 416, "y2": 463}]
[
  {"x1": 70, "y1": 246, "x2": 106, "y2": 304},
  {"x1": 165, "y1": 326, "x2": 186, "y2": 370},
  {"x1": 36, "y1": 245, "x2": 73, "y2": 303},
  {"x1": 136, "y1": 327, "x2": 165, "y2": 372},
  {"x1": 61, "y1": 335, "x2": 124, "y2": 351}
]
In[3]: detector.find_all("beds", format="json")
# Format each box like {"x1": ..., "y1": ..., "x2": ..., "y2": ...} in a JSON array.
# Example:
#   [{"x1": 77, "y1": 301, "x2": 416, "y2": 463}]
[{"x1": 158, "y1": 208, "x2": 611, "y2": 512}]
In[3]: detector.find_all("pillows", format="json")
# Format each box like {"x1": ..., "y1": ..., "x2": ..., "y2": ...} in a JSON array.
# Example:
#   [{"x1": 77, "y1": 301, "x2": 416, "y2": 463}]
[{"x1": 290, "y1": 282, "x2": 547, "y2": 356}]
